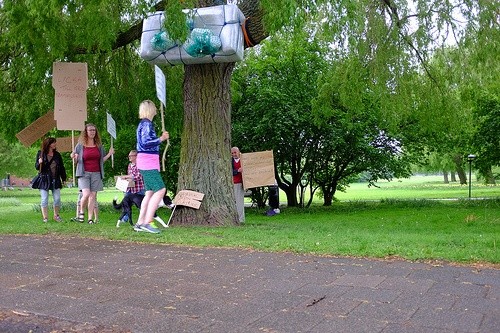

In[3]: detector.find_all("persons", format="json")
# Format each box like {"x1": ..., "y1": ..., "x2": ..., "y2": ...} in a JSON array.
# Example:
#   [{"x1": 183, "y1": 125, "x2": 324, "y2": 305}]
[
  {"x1": 32, "y1": 137, "x2": 67, "y2": 223},
  {"x1": 231, "y1": 146, "x2": 245, "y2": 224},
  {"x1": 70, "y1": 123, "x2": 115, "y2": 224},
  {"x1": 71, "y1": 189, "x2": 99, "y2": 224},
  {"x1": 133, "y1": 100, "x2": 169, "y2": 234},
  {"x1": 119, "y1": 151, "x2": 145, "y2": 223}
]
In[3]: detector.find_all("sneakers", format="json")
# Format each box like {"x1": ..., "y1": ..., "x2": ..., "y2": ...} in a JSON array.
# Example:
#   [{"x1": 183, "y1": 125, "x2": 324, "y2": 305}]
[{"x1": 134, "y1": 221, "x2": 161, "y2": 233}]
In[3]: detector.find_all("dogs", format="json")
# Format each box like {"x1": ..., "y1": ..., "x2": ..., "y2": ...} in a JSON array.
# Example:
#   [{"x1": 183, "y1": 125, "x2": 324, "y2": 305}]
[{"x1": 112, "y1": 192, "x2": 175, "y2": 230}]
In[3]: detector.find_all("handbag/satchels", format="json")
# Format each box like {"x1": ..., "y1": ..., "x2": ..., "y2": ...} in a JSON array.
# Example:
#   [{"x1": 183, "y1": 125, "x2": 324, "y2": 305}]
[{"x1": 30, "y1": 172, "x2": 42, "y2": 189}]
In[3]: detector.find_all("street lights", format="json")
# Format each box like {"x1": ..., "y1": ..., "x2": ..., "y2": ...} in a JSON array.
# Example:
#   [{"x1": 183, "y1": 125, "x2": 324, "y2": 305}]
[{"x1": 467, "y1": 154, "x2": 475, "y2": 198}]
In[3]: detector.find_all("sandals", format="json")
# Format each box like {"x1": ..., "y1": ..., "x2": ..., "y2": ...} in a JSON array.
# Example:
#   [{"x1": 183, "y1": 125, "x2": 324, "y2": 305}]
[
  {"x1": 79, "y1": 213, "x2": 84, "y2": 223},
  {"x1": 87, "y1": 220, "x2": 94, "y2": 224}
]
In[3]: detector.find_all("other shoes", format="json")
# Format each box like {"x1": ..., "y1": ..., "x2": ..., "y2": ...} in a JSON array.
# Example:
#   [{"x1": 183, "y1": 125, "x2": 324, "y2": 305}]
[
  {"x1": 42, "y1": 217, "x2": 48, "y2": 222},
  {"x1": 71, "y1": 217, "x2": 79, "y2": 221},
  {"x1": 93, "y1": 220, "x2": 100, "y2": 224},
  {"x1": 52, "y1": 215, "x2": 62, "y2": 223},
  {"x1": 121, "y1": 215, "x2": 129, "y2": 222}
]
